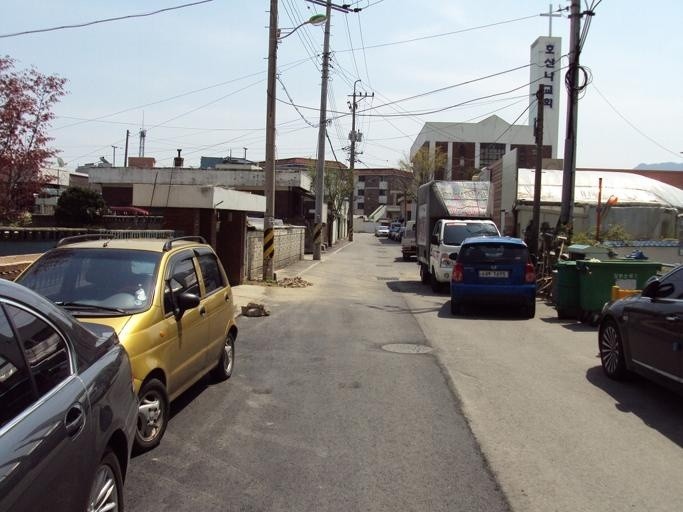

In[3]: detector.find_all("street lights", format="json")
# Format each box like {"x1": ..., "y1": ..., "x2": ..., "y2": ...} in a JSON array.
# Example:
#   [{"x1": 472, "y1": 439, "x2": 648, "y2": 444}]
[{"x1": 261, "y1": 14, "x2": 325, "y2": 282}]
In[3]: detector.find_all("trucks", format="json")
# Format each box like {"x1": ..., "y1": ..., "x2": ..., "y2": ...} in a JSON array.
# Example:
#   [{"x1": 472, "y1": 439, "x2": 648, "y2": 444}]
[{"x1": 415, "y1": 182, "x2": 502, "y2": 294}]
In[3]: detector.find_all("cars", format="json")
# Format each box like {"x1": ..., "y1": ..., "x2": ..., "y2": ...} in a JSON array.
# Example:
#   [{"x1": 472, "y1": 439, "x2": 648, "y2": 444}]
[
  {"x1": 11, "y1": 233, "x2": 237, "y2": 449},
  {"x1": 596, "y1": 264, "x2": 682, "y2": 400},
  {"x1": 400, "y1": 221, "x2": 416, "y2": 261},
  {"x1": 0, "y1": 277, "x2": 138, "y2": 511},
  {"x1": 447, "y1": 236, "x2": 536, "y2": 319},
  {"x1": 374, "y1": 223, "x2": 405, "y2": 242}
]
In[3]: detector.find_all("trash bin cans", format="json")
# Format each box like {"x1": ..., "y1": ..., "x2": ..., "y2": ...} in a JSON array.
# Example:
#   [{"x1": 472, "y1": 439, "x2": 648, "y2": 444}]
[{"x1": 551, "y1": 244, "x2": 662, "y2": 328}]
